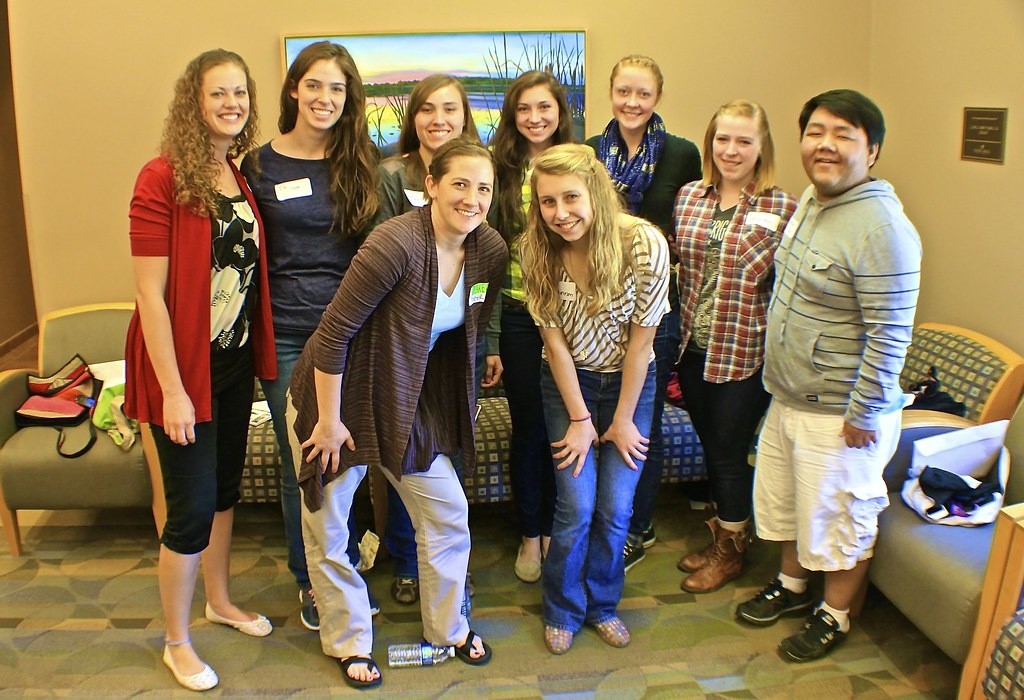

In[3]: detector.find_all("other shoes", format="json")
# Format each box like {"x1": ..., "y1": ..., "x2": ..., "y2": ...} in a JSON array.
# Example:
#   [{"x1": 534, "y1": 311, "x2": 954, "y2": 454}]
[
  {"x1": 163, "y1": 643, "x2": 219, "y2": 691},
  {"x1": 643, "y1": 522, "x2": 658, "y2": 549},
  {"x1": 593, "y1": 616, "x2": 630, "y2": 648},
  {"x1": 463, "y1": 570, "x2": 475, "y2": 598},
  {"x1": 544, "y1": 625, "x2": 574, "y2": 655},
  {"x1": 391, "y1": 576, "x2": 418, "y2": 605},
  {"x1": 515, "y1": 542, "x2": 543, "y2": 581},
  {"x1": 205, "y1": 602, "x2": 273, "y2": 637},
  {"x1": 621, "y1": 533, "x2": 646, "y2": 572}
]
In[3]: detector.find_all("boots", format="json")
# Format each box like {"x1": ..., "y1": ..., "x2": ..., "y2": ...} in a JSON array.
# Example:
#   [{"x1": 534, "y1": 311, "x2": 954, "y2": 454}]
[
  {"x1": 679, "y1": 516, "x2": 758, "y2": 574},
  {"x1": 681, "y1": 518, "x2": 751, "y2": 593}
]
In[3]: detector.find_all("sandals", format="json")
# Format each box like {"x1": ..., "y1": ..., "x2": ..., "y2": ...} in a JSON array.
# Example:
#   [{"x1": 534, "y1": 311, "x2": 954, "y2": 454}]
[
  {"x1": 450, "y1": 630, "x2": 493, "y2": 665},
  {"x1": 339, "y1": 652, "x2": 382, "y2": 688}
]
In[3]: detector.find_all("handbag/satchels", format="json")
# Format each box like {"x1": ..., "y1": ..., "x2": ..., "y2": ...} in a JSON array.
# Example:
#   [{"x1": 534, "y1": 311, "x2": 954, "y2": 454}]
[
  {"x1": 15, "y1": 354, "x2": 105, "y2": 458},
  {"x1": 92, "y1": 383, "x2": 143, "y2": 451}
]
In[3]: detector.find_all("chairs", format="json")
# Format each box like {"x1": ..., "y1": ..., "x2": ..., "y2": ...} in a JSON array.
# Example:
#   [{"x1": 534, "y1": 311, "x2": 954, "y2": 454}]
[{"x1": 0, "y1": 303, "x2": 1024, "y2": 700}]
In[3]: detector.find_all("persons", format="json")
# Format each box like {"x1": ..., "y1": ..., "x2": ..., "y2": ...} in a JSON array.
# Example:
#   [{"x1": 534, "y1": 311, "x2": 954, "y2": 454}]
[
  {"x1": 736, "y1": 89, "x2": 922, "y2": 669},
  {"x1": 241, "y1": 40, "x2": 383, "y2": 627},
  {"x1": 122, "y1": 49, "x2": 275, "y2": 692},
  {"x1": 493, "y1": 69, "x2": 576, "y2": 586},
  {"x1": 674, "y1": 98, "x2": 800, "y2": 594},
  {"x1": 585, "y1": 52, "x2": 706, "y2": 576},
  {"x1": 369, "y1": 71, "x2": 481, "y2": 606},
  {"x1": 505, "y1": 142, "x2": 673, "y2": 655},
  {"x1": 286, "y1": 136, "x2": 510, "y2": 685}
]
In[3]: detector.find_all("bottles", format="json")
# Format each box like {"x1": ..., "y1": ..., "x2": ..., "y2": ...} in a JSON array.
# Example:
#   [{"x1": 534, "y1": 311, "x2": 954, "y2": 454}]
[{"x1": 388, "y1": 643, "x2": 455, "y2": 668}]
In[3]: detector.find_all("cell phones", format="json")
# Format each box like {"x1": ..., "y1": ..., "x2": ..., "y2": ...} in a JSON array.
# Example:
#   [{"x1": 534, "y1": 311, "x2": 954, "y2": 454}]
[
  {"x1": 475, "y1": 404, "x2": 481, "y2": 421},
  {"x1": 75, "y1": 394, "x2": 95, "y2": 408}
]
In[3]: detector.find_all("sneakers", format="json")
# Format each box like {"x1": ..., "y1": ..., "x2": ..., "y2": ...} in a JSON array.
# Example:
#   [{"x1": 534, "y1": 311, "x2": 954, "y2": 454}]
[
  {"x1": 368, "y1": 587, "x2": 379, "y2": 615},
  {"x1": 779, "y1": 606, "x2": 848, "y2": 662},
  {"x1": 298, "y1": 583, "x2": 322, "y2": 631},
  {"x1": 735, "y1": 578, "x2": 815, "y2": 627}
]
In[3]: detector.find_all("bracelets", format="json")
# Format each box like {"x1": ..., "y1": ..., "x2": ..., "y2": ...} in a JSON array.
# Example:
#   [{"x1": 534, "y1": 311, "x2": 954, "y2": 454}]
[{"x1": 569, "y1": 413, "x2": 592, "y2": 423}]
